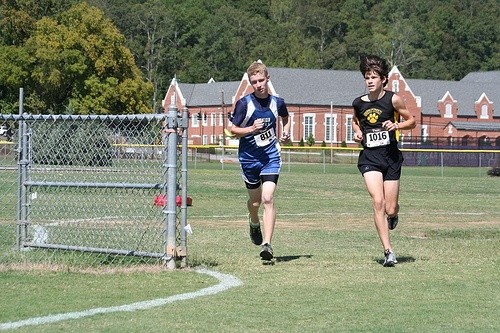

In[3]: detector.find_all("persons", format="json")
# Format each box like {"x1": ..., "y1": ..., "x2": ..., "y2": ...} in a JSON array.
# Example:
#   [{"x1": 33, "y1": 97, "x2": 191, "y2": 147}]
[
  {"x1": 227, "y1": 63, "x2": 291, "y2": 260},
  {"x1": 352, "y1": 55, "x2": 416, "y2": 264}
]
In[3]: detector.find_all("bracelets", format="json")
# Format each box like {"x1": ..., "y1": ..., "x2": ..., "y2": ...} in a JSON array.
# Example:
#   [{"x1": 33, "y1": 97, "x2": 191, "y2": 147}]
[{"x1": 396, "y1": 122, "x2": 399, "y2": 130}]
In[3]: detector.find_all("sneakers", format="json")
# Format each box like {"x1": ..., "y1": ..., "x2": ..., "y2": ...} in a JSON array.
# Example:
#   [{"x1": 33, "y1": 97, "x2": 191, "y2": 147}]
[
  {"x1": 248, "y1": 214, "x2": 262, "y2": 245},
  {"x1": 383, "y1": 249, "x2": 398, "y2": 266},
  {"x1": 387, "y1": 216, "x2": 398, "y2": 230},
  {"x1": 259, "y1": 243, "x2": 273, "y2": 261}
]
